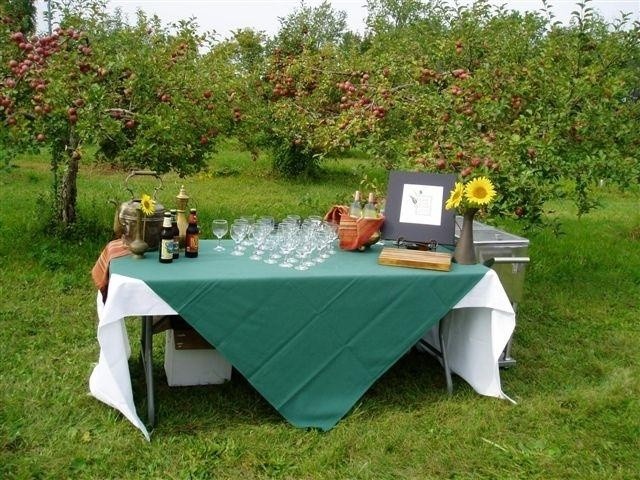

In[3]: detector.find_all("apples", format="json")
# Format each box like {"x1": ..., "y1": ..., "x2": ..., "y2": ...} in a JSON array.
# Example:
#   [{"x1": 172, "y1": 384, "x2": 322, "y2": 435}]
[{"x1": 0, "y1": 12, "x2": 553, "y2": 217}]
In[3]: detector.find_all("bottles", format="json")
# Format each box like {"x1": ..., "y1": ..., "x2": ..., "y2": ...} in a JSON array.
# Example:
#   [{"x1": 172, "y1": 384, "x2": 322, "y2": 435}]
[
  {"x1": 185, "y1": 208, "x2": 199, "y2": 258},
  {"x1": 159, "y1": 212, "x2": 174, "y2": 263},
  {"x1": 364, "y1": 192, "x2": 377, "y2": 218},
  {"x1": 169, "y1": 208, "x2": 180, "y2": 260},
  {"x1": 349, "y1": 190, "x2": 361, "y2": 220}
]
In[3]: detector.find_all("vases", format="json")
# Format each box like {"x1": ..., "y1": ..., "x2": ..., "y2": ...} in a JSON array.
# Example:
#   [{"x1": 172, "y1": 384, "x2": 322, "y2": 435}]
[
  {"x1": 451, "y1": 206, "x2": 478, "y2": 265},
  {"x1": 129, "y1": 208, "x2": 148, "y2": 258}
]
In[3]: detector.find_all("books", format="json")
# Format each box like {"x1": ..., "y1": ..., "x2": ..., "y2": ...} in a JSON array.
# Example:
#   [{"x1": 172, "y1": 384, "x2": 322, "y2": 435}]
[{"x1": 378, "y1": 247, "x2": 453, "y2": 271}]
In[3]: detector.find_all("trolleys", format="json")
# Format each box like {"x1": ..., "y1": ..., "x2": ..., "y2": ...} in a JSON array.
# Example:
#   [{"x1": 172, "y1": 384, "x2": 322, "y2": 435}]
[{"x1": 439, "y1": 214, "x2": 532, "y2": 371}]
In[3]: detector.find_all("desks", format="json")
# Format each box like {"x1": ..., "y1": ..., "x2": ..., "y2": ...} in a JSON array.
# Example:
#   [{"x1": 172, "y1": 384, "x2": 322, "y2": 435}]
[{"x1": 86, "y1": 238, "x2": 517, "y2": 440}]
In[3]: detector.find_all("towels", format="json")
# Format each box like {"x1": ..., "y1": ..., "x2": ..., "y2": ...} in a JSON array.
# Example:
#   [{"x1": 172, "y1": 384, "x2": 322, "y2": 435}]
[
  {"x1": 91, "y1": 237, "x2": 134, "y2": 303},
  {"x1": 323, "y1": 204, "x2": 385, "y2": 251}
]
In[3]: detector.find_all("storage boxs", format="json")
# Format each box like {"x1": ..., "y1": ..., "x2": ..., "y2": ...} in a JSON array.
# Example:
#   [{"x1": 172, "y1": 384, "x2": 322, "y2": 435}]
[{"x1": 163, "y1": 328, "x2": 232, "y2": 387}]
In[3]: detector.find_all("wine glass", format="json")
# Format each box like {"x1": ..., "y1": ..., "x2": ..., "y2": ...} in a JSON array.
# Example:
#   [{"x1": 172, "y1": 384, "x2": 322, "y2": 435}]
[{"x1": 212, "y1": 214, "x2": 339, "y2": 270}]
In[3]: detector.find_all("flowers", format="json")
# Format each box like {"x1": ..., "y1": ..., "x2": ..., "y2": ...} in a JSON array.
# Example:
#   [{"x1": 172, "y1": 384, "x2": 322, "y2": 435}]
[
  {"x1": 139, "y1": 193, "x2": 156, "y2": 216},
  {"x1": 445, "y1": 176, "x2": 497, "y2": 214}
]
